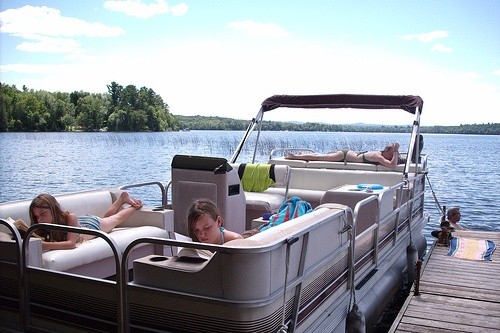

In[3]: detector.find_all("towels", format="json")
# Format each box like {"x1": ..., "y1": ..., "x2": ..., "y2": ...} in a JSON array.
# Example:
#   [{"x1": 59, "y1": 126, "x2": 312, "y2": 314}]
[
  {"x1": 255, "y1": 196, "x2": 313, "y2": 232},
  {"x1": 444, "y1": 237, "x2": 496, "y2": 261}
]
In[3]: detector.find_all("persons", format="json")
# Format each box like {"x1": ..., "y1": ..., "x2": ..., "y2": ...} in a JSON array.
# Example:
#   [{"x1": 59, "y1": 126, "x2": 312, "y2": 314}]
[
  {"x1": 447, "y1": 207, "x2": 468, "y2": 230},
  {"x1": 187, "y1": 196, "x2": 309, "y2": 253},
  {"x1": 29, "y1": 192, "x2": 143, "y2": 250},
  {"x1": 284, "y1": 143, "x2": 400, "y2": 168}
]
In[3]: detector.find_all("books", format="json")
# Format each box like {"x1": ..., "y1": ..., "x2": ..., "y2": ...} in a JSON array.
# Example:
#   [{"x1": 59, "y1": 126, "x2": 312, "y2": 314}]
[
  {"x1": 173, "y1": 247, "x2": 214, "y2": 262},
  {"x1": 7, "y1": 217, "x2": 45, "y2": 240}
]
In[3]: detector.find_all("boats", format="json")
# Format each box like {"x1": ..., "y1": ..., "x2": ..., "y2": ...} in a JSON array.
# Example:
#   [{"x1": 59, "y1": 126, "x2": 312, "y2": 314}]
[{"x1": 0, "y1": 94, "x2": 431, "y2": 333}]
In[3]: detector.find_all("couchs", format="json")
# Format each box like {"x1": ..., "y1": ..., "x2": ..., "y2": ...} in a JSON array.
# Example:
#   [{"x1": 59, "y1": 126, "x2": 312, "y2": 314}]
[
  {"x1": 0, "y1": 188, "x2": 178, "y2": 279},
  {"x1": 131, "y1": 203, "x2": 349, "y2": 301}
]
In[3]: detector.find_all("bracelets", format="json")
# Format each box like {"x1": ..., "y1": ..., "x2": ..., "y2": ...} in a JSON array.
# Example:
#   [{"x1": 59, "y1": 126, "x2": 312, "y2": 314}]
[{"x1": 393, "y1": 150, "x2": 398, "y2": 152}]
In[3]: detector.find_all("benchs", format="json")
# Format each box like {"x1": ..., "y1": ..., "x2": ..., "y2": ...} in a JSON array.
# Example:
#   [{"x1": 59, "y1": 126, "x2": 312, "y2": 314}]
[
  {"x1": 268, "y1": 148, "x2": 425, "y2": 201},
  {"x1": 228, "y1": 163, "x2": 290, "y2": 225}
]
